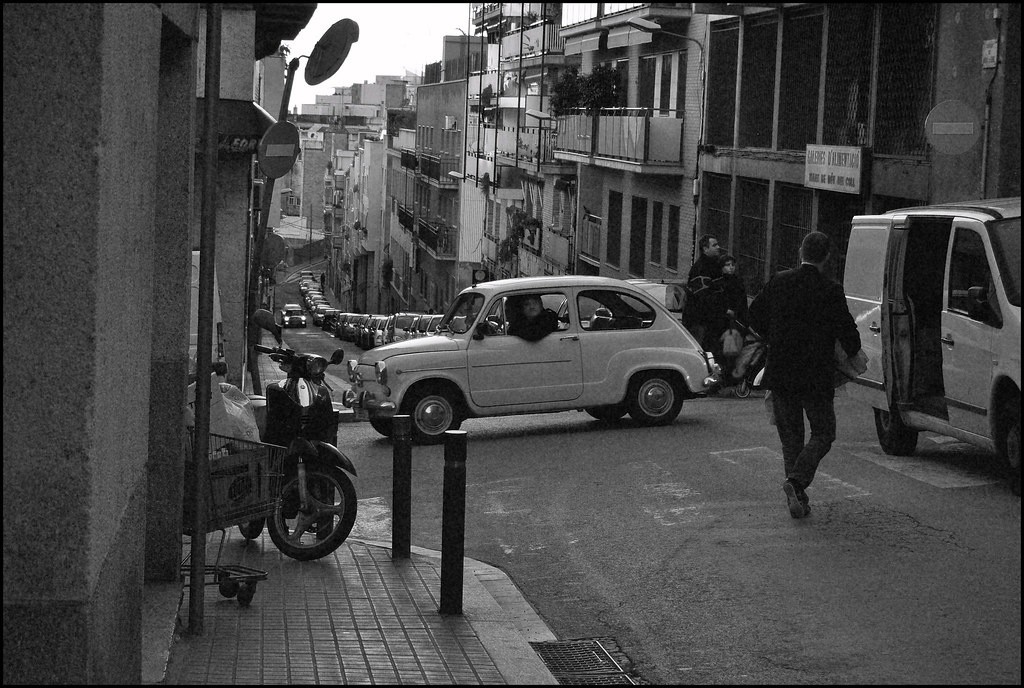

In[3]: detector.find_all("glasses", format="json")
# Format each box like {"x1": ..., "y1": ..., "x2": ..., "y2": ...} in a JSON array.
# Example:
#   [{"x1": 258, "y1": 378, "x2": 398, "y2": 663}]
[
  {"x1": 723, "y1": 262, "x2": 737, "y2": 266},
  {"x1": 712, "y1": 241, "x2": 722, "y2": 247}
]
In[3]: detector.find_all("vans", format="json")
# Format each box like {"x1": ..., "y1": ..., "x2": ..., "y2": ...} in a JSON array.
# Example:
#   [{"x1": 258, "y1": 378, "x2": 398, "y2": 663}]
[{"x1": 838, "y1": 197, "x2": 1023, "y2": 496}]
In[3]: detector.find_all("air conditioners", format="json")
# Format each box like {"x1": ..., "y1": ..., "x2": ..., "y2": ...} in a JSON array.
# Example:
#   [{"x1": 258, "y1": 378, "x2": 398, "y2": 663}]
[
  {"x1": 334, "y1": 173, "x2": 344, "y2": 188},
  {"x1": 445, "y1": 116, "x2": 455, "y2": 130}
]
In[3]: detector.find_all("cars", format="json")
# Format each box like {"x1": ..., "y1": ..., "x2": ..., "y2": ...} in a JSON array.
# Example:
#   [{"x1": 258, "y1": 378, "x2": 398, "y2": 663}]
[
  {"x1": 342, "y1": 276, "x2": 722, "y2": 445},
  {"x1": 279, "y1": 269, "x2": 451, "y2": 348},
  {"x1": 623, "y1": 278, "x2": 688, "y2": 325}
]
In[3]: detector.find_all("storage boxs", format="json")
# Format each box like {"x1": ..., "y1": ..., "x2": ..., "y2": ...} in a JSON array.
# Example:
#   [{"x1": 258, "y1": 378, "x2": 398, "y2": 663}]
[{"x1": 186, "y1": 370, "x2": 234, "y2": 455}]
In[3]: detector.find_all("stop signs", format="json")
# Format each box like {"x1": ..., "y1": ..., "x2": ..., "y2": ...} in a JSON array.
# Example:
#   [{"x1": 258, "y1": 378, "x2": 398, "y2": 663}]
[
  {"x1": 257, "y1": 121, "x2": 299, "y2": 178},
  {"x1": 925, "y1": 99, "x2": 982, "y2": 154}
]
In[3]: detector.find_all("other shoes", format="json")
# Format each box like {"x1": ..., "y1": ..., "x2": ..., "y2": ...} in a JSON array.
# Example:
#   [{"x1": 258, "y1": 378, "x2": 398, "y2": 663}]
[{"x1": 783, "y1": 478, "x2": 811, "y2": 519}]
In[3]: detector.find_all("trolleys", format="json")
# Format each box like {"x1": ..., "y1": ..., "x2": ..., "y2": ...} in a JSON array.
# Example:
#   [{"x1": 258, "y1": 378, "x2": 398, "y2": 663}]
[{"x1": 180, "y1": 425, "x2": 288, "y2": 610}]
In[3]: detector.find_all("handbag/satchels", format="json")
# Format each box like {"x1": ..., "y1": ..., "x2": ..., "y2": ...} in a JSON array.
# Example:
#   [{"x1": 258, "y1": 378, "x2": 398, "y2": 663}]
[{"x1": 718, "y1": 317, "x2": 743, "y2": 357}]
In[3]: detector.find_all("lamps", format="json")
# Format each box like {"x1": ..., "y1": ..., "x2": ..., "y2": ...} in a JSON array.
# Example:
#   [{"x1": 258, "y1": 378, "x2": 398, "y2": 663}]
[
  {"x1": 525, "y1": 109, "x2": 556, "y2": 121},
  {"x1": 449, "y1": 171, "x2": 465, "y2": 181},
  {"x1": 626, "y1": 16, "x2": 704, "y2": 67}
]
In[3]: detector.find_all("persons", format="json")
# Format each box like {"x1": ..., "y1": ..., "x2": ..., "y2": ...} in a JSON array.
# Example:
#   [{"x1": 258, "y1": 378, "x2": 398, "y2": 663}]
[
  {"x1": 749, "y1": 231, "x2": 861, "y2": 516},
  {"x1": 681, "y1": 232, "x2": 747, "y2": 370},
  {"x1": 509, "y1": 295, "x2": 558, "y2": 342}
]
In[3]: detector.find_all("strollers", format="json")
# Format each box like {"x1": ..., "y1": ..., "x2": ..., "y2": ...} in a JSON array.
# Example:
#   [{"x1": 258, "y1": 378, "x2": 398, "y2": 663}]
[{"x1": 733, "y1": 319, "x2": 769, "y2": 399}]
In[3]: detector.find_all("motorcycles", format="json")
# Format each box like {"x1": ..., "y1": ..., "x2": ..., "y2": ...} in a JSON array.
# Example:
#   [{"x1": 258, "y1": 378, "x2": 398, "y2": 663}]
[{"x1": 239, "y1": 309, "x2": 359, "y2": 562}]
